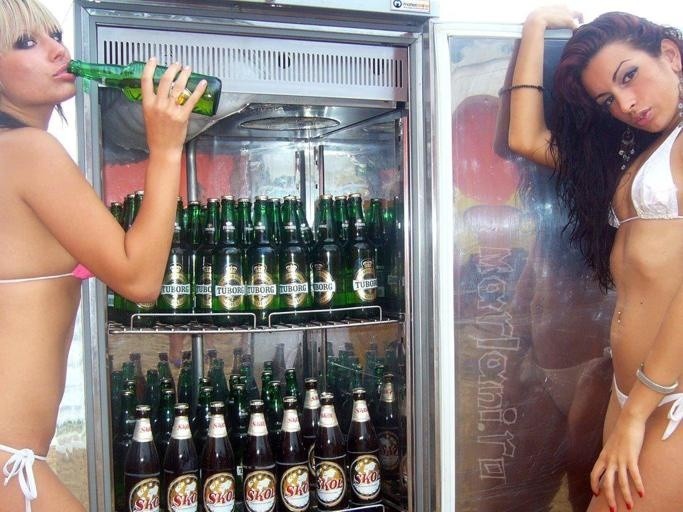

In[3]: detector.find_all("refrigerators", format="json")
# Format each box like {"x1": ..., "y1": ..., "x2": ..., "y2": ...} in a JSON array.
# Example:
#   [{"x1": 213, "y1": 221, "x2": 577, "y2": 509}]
[{"x1": 73, "y1": 2, "x2": 614, "y2": 512}]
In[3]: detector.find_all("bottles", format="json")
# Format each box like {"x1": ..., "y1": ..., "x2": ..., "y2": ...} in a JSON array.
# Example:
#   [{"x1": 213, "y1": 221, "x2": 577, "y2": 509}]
[
  {"x1": 364, "y1": 196, "x2": 406, "y2": 313},
  {"x1": 105, "y1": 341, "x2": 407, "y2": 512},
  {"x1": 107, "y1": 188, "x2": 387, "y2": 325},
  {"x1": 67, "y1": 58, "x2": 222, "y2": 118}
]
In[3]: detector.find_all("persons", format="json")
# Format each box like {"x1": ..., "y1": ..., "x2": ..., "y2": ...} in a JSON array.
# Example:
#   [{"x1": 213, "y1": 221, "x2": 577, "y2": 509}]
[
  {"x1": 507, "y1": 10, "x2": 683, "y2": 512},
  {"x1": 0, "y1": 1, "x2": 210, "y2": 512}
]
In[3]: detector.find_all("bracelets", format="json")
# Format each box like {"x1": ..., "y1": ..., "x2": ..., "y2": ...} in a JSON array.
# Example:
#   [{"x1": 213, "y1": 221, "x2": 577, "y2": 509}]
[
  {"x1": 636, "y1": 360, "x2": 678, "y2": 395},
  {"x1": 509, "y1": 85, "x2": 545, "y2": 91}
]
